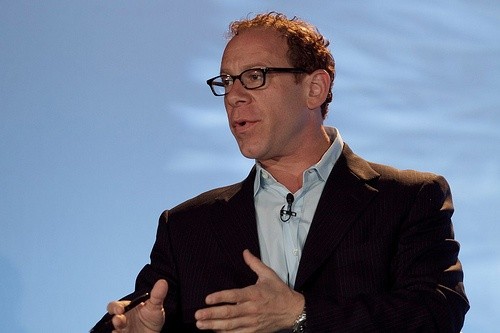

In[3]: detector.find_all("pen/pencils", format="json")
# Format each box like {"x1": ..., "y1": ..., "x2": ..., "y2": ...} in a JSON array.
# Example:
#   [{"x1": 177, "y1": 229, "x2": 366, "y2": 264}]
[{"x1": 90, "y1": 290, "x2": 150, "y2": 333}]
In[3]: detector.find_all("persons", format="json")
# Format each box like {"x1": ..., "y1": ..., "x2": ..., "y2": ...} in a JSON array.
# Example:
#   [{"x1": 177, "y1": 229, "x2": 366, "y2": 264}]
[{"x1": 87, "y1": 10, "x2": 469, "y2": 333}]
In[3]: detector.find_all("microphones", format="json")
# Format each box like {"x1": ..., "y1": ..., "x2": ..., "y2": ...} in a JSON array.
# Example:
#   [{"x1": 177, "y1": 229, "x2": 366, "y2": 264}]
[{"x1": 280, "y1": 193, "x2": 294, "y2": 222}]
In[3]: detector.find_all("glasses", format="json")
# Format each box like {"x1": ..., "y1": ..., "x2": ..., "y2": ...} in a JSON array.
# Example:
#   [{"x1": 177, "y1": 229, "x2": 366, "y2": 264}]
[{"x1": 206, "y1": 67, "x2": 307, "y2": 97}]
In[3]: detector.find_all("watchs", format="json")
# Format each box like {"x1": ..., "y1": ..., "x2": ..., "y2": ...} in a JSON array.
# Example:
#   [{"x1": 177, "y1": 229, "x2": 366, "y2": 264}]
[{"x1": 291, "y1": 302, "x2": 306, "y2": 333}]
